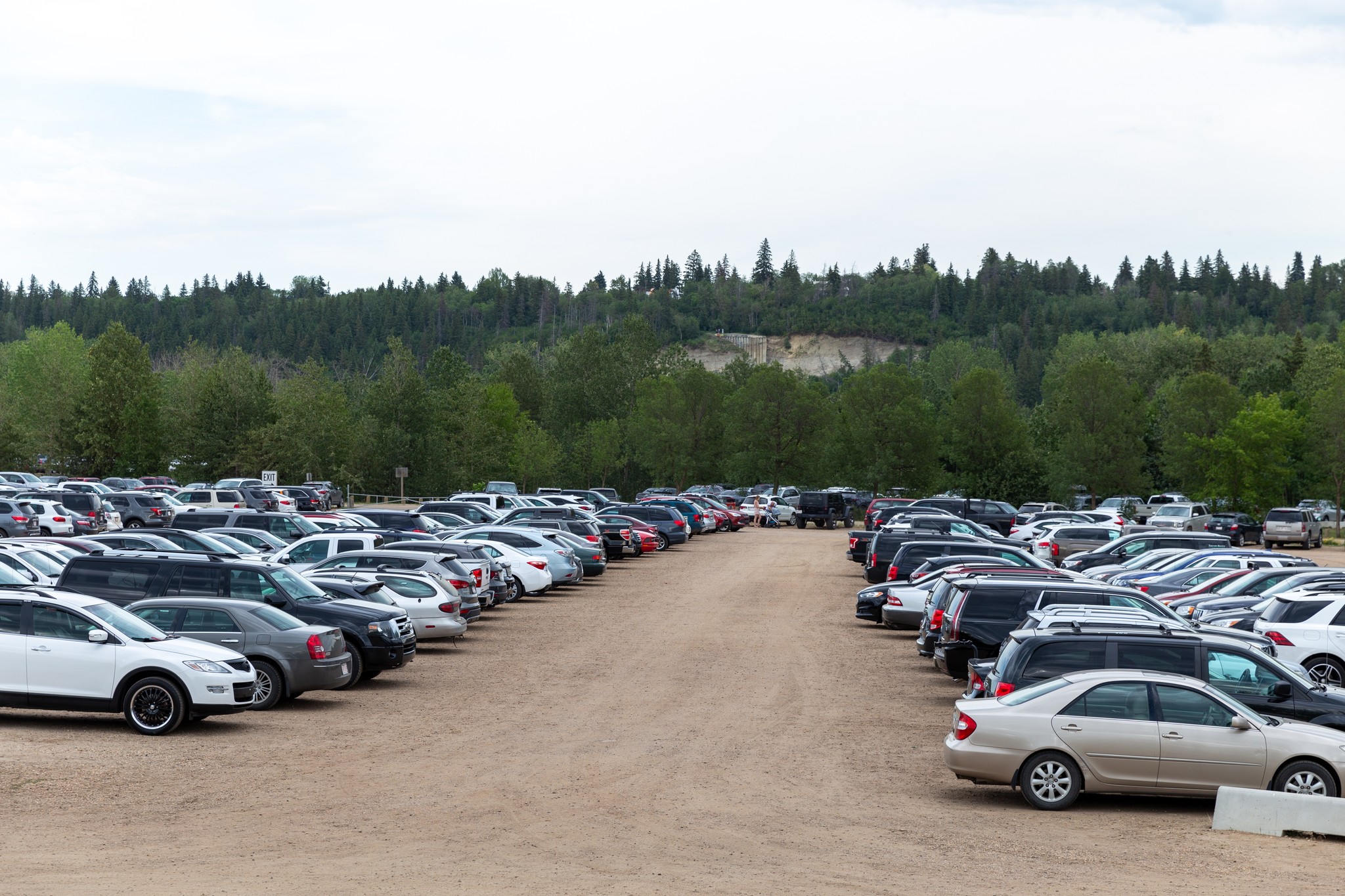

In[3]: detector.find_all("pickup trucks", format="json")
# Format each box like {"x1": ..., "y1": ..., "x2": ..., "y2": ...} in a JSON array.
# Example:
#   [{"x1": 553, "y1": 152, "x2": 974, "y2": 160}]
[{"x1": 1132, "y1": 494, "x2": 1191, "y2": 525}]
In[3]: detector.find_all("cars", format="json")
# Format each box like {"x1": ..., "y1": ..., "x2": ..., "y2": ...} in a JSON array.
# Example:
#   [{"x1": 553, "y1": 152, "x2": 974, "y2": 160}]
[
  {"x1": 944, "y1": 669, "x2": 1345, "y2": 812},
  {"x1": 87, "y1": 595, "x2": 353, "y2": 711}
]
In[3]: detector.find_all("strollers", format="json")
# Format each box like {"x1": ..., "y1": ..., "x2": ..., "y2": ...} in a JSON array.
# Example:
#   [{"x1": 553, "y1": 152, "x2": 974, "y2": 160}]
[{"x1": 763, "y1": 506, "x2": 782, "y2": 529}]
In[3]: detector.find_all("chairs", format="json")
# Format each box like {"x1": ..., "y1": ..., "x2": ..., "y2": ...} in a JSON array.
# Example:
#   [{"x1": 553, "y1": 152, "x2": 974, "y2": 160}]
[
  {"x1": 200, "y1": 614, "x2": 214, "y2": 631},
  {"x1": 1124, "y1": 691, "x2": 1134, "y2": 718},
  {"x1": 33, "y1": 610, "x2": 52, "y2": 637},
  {"x1": 1130, "y1": 694, "x2": 1148, "y2": 720},
  {"x1": 276, "y1": 518, "x2": 287, "y2": 537},
  {"x1": 231, "y1": 571, "x2": 238, "y2": 599},
  {"x1": 47, "y1": 612, "x2": 73, "y2": 639},
  {"x1": 269, "y1": 518, "x2": 275, "y2": 535},
  {"x1": 237, "y1": 571, "x2": 257, "y2": 601}
]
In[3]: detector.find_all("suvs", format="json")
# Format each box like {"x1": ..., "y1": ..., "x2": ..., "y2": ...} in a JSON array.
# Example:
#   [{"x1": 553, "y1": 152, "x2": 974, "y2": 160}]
[
  {"x1": 1254, "y1": 586, "x2": 1345, "y2": 690},
  {"x1": 0, "y1": 583, "x2": 258, "y2": 736},
  {"x1": 1204, "y1": 511, "x2": 1264, "y2": 547},
  {"x1": 983, "y1": 619, "x2": 1344, "y2": 732},
  {"x1": 761, "y1": 486, "x2": 802, "y2": 510},
  {"x1": 1146, "y1": 501, "x2": 1212, "y2": 532},
  {"x1": 1295, "y1": 499, "x2": 1345, "y2": 522},
  {"x1": 0, "y1": 472, "x2": 775, "y2": 638},
  {"x1": 846, "y1": 487, "x2": 1345, "y2": 700},
  {"x1": 818, "y1": 487, "x2": 874, "y2": 514},
  {"x1": 53, "y1": 547, "x2": 417, "y2": 690},
  {"x1": 796, "y1": 491, "x2": 855, "y2": 530},
  {"x1": 739, "y1": 493, "x2": 797, "y2": 526},
  {"x1": 1263, "y1": 507, "x2": 1322, "y2": 550}
]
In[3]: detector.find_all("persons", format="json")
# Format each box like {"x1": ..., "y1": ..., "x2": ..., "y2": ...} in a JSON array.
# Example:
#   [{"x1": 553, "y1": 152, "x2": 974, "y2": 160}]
[
  {"x1": 754, "y1": 495, "x2": 764, "y2": 527},
  {"x1": 765, "y1": 501, "x2": 777, "y2": 528}
]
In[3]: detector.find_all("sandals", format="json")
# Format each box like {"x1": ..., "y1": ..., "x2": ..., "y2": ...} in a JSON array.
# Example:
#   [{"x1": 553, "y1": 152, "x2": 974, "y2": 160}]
[
  {"x1": 754, "y1": 526, "x2": 758, "y2": 527},
  {"x1": 757, "y1": 525, "x2": 762, "y2": 528}
]
